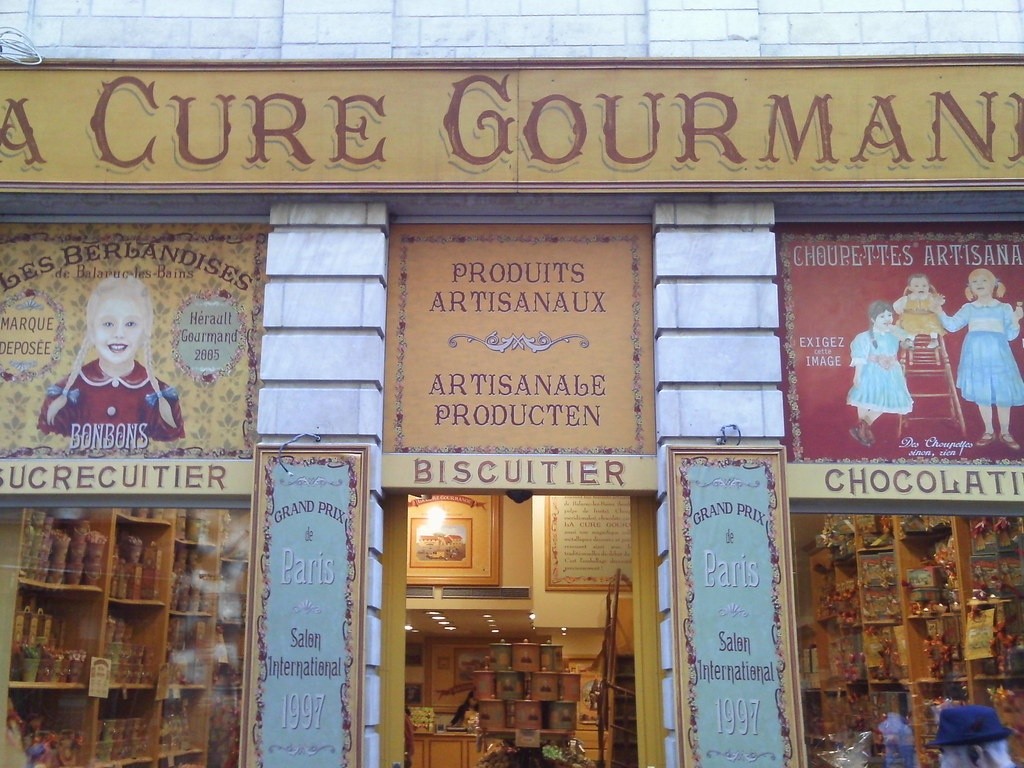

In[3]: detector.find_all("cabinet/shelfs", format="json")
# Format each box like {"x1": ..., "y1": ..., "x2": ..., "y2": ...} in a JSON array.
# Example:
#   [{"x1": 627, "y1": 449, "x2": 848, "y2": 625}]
[
  {"x1": 410, "y1": 731, "x2": 609, "y2": 768},
  {"x1": 792, "y1": 515, "x2": 1024, "y2": 768},
  {"x1": 0, "y1": 507, "x2": 251, "y2": 768}
]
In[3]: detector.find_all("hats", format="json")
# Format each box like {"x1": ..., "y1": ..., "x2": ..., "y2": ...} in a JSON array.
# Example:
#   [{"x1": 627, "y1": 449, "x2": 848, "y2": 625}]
[{"x1": 927, "y1": 705, "x2": 1012, "y2": 747}]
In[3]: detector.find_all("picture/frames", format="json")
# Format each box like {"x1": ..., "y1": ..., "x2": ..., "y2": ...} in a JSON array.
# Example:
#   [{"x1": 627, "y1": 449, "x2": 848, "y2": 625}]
[
  {"x1": 667, "y1": 446, "x2": 804, "y2": 768},
  {"x1": 423, "y1": 638, "x2": 496, "y2": 712},
  {"x1": 545, "y1": 495, "x2": 632, "y2": 591},
  {"x1": 406, "y1": 494, "x2": 500, "y2": 585}
]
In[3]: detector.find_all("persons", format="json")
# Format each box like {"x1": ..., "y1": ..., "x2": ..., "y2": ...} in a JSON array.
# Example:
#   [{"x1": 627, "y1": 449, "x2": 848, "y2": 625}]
[
  {"x1": 447, "y1": 685, "x2": 480, "y2": 727},
  {"x1": 878, "y1": 694, "x2": 916, "y2": 768},
  {"x1": 926, "y1": 706, "x2": 1024, "y2": 768},
  {"x1": 404, "y1": 705, "x2": 414, "y2": 768}
]
